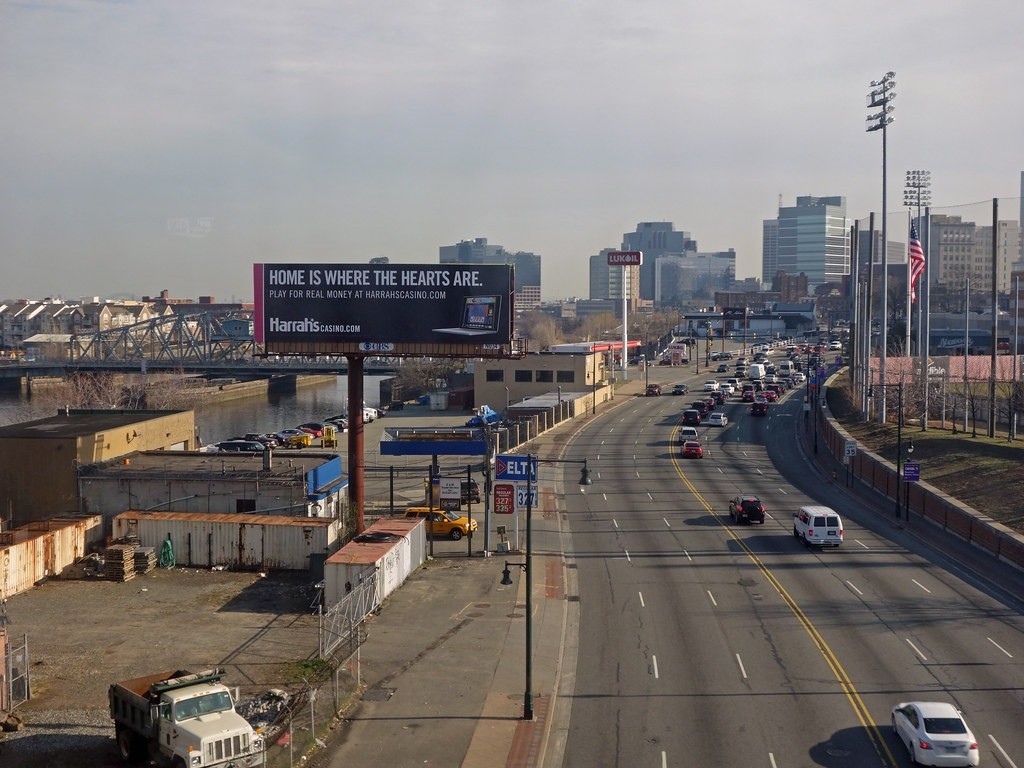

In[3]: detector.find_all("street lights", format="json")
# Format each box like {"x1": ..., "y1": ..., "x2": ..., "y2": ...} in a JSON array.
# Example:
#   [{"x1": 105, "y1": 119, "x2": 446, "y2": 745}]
[
  {"x1": 592, "y1": 342, "x2": 612, "y2": 417},
  {"x1": 524, "y1": 455, "x2": 595, "y2": 721},
  {"x1": 867, "y1": 382, "x2": 903, "y2": 519},
  {"x1": 645, "y1": 332, "x2": 660, "y2": 396}
]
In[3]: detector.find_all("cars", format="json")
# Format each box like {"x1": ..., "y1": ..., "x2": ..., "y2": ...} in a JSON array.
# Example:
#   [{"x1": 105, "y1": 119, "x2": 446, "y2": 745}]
[
  {"x1": 791, "y1": 506, "x2": 844, "y2": 545},
  {"x1": 891, "y1": 700, "x2": 982, "y2": 767},
  {"x1": 405, "y1": 506, "x2": 479, "y2": 540},
  {"x1": 217, "y1": 440, "x2": 270, "y2": 452},
  {"x1": 680, "y1": 440, "x2": 705, "y2": 458},
  {"x1": 678, "y1": 426, "x2": 699, "y2": 442},
  {"x1": 729, "y1": 495, "x2": 765, "y2": 524},
  {"x1": 692, "y1": 329, "x2": 877, "y2": 417},
  {"x1": 658, "y1": 338, "x2": 694, "y2": 366},
  {"x1": 683, "y1": 409, "x2": 701, "y2": 425},
  {"x1": 243, "y1": 407, "x2": 389, "y2": 448},
  {"x1": 708, "y1": 412, "x2": 728, "y2": 428},
  {"x1": 671, "y1": 383, "x2": 688, "y2": 395},
  {"x1": 646, "y1": 384, "x2": 661, "y2": 396},
  {"x1": 461, "y1": 477, "x2": 481, "y2": 505}
]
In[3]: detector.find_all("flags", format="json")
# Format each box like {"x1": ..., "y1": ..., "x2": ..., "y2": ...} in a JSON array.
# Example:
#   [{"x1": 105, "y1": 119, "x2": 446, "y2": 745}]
[{"x1": 908, "y1": 216, "x2": 926, "y2": 304}]
